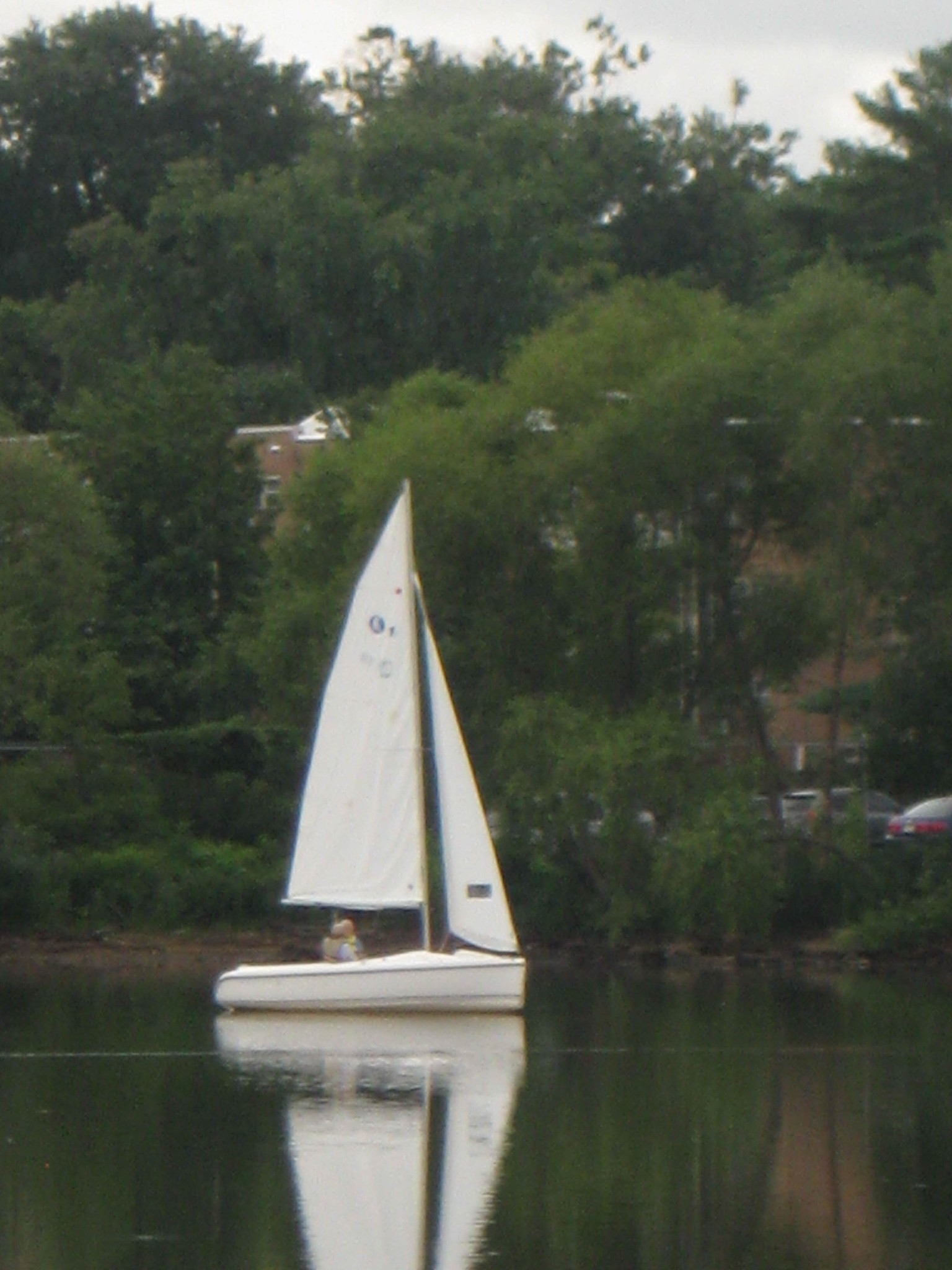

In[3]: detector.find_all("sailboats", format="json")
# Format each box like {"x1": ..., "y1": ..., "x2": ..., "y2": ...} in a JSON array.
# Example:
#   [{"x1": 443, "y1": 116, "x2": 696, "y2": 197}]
[{"x1": 216, "y1": 473, "x2": 532, "y2": 1016}]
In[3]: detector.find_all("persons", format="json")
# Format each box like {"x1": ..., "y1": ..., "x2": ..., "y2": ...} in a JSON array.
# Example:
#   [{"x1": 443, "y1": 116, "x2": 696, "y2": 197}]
[{"x1": 321, "y1": 920, "x2": 364, "y2": 961}]
